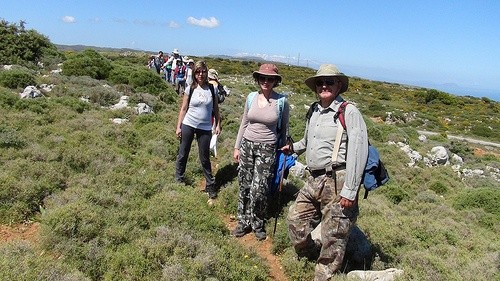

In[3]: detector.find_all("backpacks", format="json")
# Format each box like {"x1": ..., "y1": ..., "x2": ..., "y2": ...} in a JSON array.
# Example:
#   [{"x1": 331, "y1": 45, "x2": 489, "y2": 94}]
[{"x1": 213, "y1": 81, "x2": 226, "y2": 104}]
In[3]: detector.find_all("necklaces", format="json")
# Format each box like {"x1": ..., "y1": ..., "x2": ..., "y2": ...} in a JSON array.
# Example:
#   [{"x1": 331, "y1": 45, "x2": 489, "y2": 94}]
[{"x1": 262, "y1": 88, "x2": 272, "y2": 106}]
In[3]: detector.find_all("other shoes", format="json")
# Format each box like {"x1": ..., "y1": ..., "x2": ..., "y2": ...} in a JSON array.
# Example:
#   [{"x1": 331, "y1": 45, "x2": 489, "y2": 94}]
[
  {"x1": 233, "y1": 226, "x2": 246, "y2": 237},
  {"x1": 254, "y1": 227, "x2": 266, "y2": 240}
]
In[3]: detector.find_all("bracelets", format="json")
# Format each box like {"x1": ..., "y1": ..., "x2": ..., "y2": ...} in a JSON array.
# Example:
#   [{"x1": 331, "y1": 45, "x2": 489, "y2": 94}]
[{"x1": 288, "y1": 143, "x2": 294, "y2": 154}]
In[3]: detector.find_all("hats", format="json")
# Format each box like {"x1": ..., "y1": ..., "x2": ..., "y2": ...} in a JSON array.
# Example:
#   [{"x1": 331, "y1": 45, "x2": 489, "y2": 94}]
[
  {"x1": 182, "y1": 56, "x2": 189, "y2": 62},
  {"x1": 208, "y1": 133, "x2": 219, "y2": 160},
  {"x1": 186, "y1": 59, "x2": 195, "y2": 65},
  {"x1": 171, "y1": 49, "x2": 179, "y2": 54},
  {"x1": 159, "y1": 51, "x2": 163, "y2": 54},
  {"x1": 252, "y1": 64, "x2": 282, "y2": 82},
  {"x1": 207, "y1": 68, "x2": 218, "y2": 80},
  {"x1": 304, "y1": 64, "x2": 349, "y2": 95},
  {"x1": 176, "y1": 59, "x2": 182, "y2": 64}
]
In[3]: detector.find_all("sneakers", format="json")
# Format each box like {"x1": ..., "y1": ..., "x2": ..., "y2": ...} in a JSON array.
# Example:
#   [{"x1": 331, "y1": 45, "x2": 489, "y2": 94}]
[
  {"x1": 209, "y1": 191, "x2": 218, "y2": 200},
  {"x1": 178, "y1": 181, "x2": 187, "y2": 186}
]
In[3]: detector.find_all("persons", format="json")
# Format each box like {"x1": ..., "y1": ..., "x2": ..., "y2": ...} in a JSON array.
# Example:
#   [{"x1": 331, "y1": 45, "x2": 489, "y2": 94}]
[
  {"x1": 207, "y1": 69, "x2": 227, "y2": 131},
  {"x1": 175, "y1": 61, "x2": 221, "y2": 198},
  {"x1": 149, "y1": 49, "x2": 195, "y2": 95},
  {"x1": 233, "y1": 64, "x2": 289, "y2": 241},
  {"x1": 281, "y1": 64, "x2": 369, "y2": 281}
]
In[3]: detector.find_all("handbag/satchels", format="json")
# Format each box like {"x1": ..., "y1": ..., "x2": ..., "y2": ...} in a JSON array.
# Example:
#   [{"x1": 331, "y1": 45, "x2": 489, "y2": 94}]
[{"x1": 360, "y1": 146, "x2": 389, "y2": 190}]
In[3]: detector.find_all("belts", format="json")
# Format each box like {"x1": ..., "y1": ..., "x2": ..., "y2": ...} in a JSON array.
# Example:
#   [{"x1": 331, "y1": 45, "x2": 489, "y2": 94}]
[{"x1": 308, "y1": 162, "x2": 347, "y2": 178}]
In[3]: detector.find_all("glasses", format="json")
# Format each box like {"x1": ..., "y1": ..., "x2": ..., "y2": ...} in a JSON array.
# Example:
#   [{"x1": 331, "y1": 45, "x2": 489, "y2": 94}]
[
  {"x1": 175, "y1": 54, "x2": 178, "y2": 55},
  {"x1": 195, "y1": 70, "x2": 206, "y2": 74},
  {"x1": 315, "y1": 78, "x2": 335, "y2": 87},
  {"x1": 256, "y1": 75, "x2": 275, "y2": 82}
]
